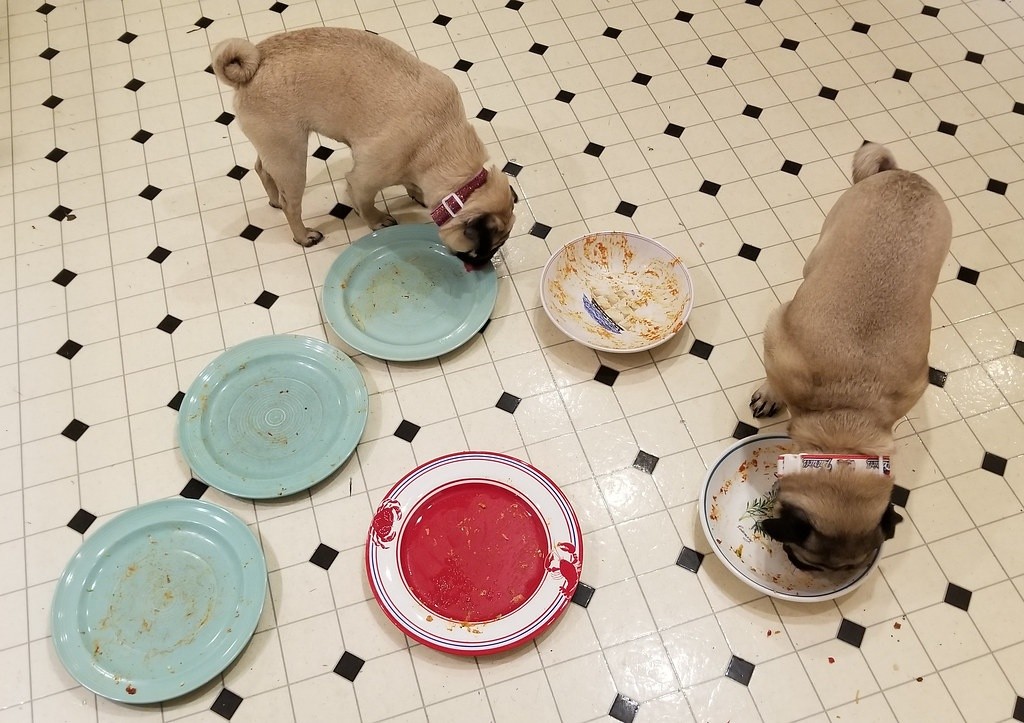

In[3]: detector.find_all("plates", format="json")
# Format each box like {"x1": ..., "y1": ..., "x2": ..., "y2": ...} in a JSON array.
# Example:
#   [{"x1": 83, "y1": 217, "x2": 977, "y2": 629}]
[
  {"x1": 698, "y1": 433, "x2": 882, "y2": 602},
  {"x1": 539, "y1": 231, "x2": 693, "y2": 354},
  {"x1": 178, "y1": 334, "x2": 374, "y2": 497},
  {"x1": 365, "y1": 451, "x2": 583, "y2": 656},
  {"x1": 322, "y1": 223, "x2": 498, "y2": 362},
  {"x1": 51, "y1": 498, "x2": 269, "y2": 705}
]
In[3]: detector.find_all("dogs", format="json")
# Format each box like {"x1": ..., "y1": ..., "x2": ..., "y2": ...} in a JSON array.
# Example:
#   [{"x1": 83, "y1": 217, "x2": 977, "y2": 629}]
[
  {"x1": 211, "y1": 28, "x2": 516, "y2": 270},
  {"x1": 749, "y1": 142, "x2": 953, "y2": 578}
]
